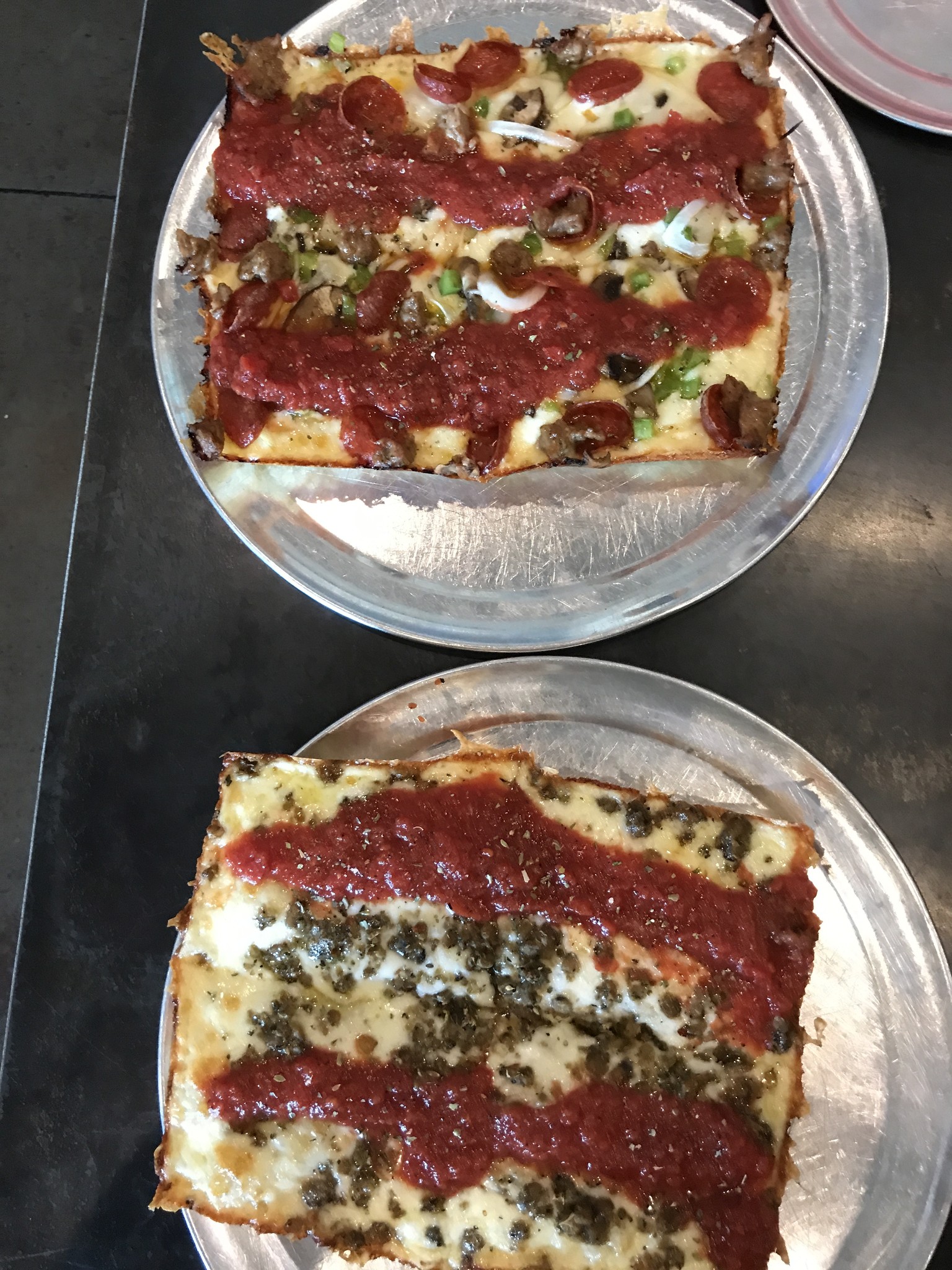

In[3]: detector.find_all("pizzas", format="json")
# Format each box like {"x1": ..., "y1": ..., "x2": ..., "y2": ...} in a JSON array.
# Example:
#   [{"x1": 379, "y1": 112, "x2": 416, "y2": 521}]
[
  {"x1": 189, "y1": 11, "x2": 799, "y2": 484},
  {"x1": 143, "y1": 740, "x2": 818, "y2": 1270}
]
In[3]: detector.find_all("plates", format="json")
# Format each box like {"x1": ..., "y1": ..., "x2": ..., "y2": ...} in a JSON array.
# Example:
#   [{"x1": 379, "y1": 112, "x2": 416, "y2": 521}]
[
  {"x1": 766, "y1": 0, "x2": 952, "y2": 136},
  {"x1": 150, "y1": 0, "x2": 891, "y2": 653},
  {"x1": 159, "y1": 656, "x2": 951, "y2": 1270}
]
